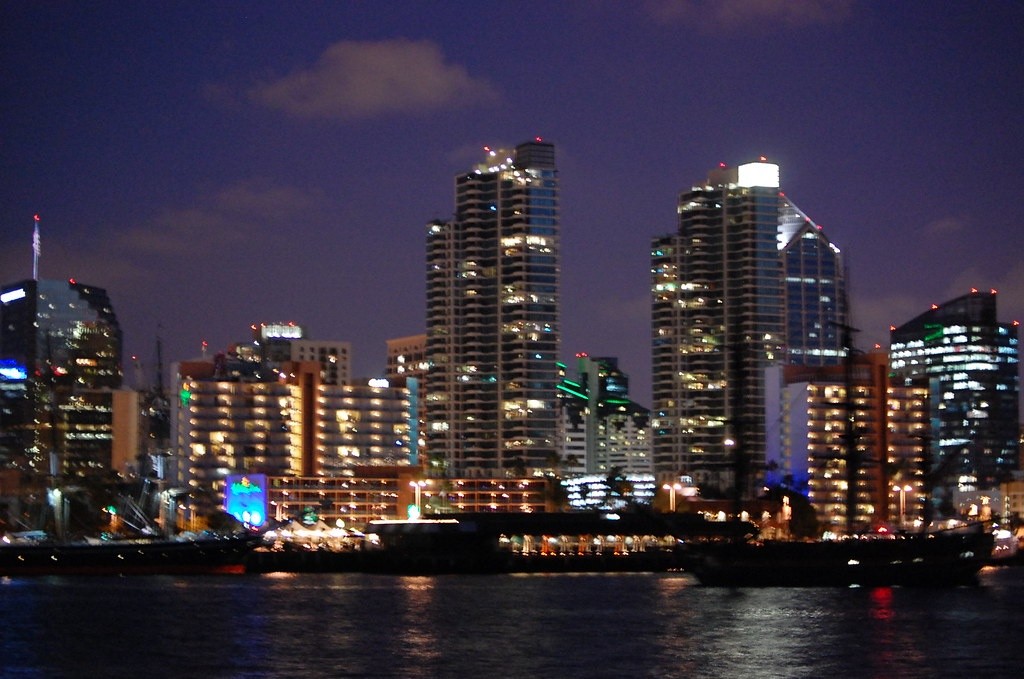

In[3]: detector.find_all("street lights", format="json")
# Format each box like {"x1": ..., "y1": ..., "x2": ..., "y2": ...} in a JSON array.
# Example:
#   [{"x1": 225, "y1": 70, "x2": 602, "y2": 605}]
[
  {"x1": 661, "y1": 483, "x2": 682, "y2": 512},
  {"x1": 892, "y1": 485, "x2": 912, "y2": 524},
  {"x1": 409, "y1": 479, "x2": 426, "y2": 510}
]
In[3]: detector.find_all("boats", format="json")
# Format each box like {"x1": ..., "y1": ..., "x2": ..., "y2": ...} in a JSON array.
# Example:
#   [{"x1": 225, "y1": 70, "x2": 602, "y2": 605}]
[
  {"x1": 673, "y1": 527, "x2": 998, "y2": 589},
  {"x1": 362, "y1": 506, "x2": 762, "y2": 577},
  {"x1": 0, "y1": 495, "x2": 334, "y2": 576}
]
